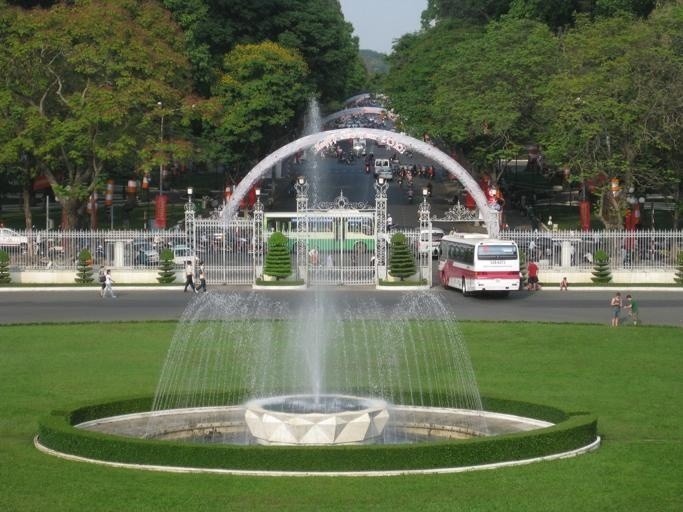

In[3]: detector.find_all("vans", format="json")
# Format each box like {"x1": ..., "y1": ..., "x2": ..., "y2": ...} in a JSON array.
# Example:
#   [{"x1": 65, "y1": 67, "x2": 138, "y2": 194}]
[
  {"x1": 414, "y1": 226, "x2": 446, "y2": 258},
  {"x1": 374, "y1": 158, "x2": 390, "y2": 176}
]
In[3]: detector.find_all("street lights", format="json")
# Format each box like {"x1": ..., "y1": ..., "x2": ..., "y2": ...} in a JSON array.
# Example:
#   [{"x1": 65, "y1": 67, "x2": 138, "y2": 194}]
[
  {"x1": 622, "y1": 187, "x2": 647, "y2": 262},
  {"x1": 156, "y1": 100, "x2": 197, "y2": 195},
  {"x1": 574, "y1": 95, "x2": 612, "y2": 181}
]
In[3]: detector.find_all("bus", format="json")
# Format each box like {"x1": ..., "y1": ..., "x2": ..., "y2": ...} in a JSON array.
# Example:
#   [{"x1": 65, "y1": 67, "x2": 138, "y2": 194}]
[
  {"x1": 263, "y1": 210, "x2": 383, "y2": 254},
  {"x1": 437, "y1": 231, "x2": 521, "y2": 298}
]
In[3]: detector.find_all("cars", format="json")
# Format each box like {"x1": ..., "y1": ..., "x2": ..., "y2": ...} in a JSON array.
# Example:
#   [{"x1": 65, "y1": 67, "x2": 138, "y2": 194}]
[
  {"x1": 335, "y1": 114, "x2": 386, "y2": 129},
  {"x1": 0, "y1": 227, "x2": 263, "y2": 270},
  {"x1": 377, "y1": 168, "x2": 394, "y2": 180},
  {"x1": 505, "y1": 235, "x2": 682, "y2": 268},
  {"x1": 374, "y1": 141, "x2": 386, "y2": 147}
]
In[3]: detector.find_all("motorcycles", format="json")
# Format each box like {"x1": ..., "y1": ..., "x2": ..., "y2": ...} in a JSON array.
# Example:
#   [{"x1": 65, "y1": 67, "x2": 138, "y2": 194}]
[{"x1": 398, "y1": 172, "x2": 433, "y2": 203}]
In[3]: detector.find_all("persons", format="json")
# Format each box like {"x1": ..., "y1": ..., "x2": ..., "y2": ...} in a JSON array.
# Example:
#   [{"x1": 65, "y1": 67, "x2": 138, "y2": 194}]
[
  {"x1": 528, "y1": 239, "x2": 632, "y2": 270},
  {"x1": 195, "y1": 261, "x2": 209, "y2": 294},
  {"x1": 137, "y1": 250, "x2": 149, "y2": 267},
  {"x1": 99, "y1": 266, "x2": 106, "y2": 290},
  {"x1": 611, "y1": 292, "x2": 622, "y2": 327},
  {"x1": 319, "y1": 101, "x2": 475, "y2": 205},
  {"x1": 183, "y1": 260, "x2": 196, "y2": 292},
  {"x1": 624, "y1": 295, "x2": 638, "y2": 327},
  {"x1": 103, "y1": 270, "x2": 117, "y2": 298},
  {"x1": 519, "y1": 259, "x2": 541, "y2": 291},
  {"x1": 383, "y1": 213, "x2": 393, "y2": 232},
  {"x1": 560, "y1": 277, "x2": 568, "y2": 292}
]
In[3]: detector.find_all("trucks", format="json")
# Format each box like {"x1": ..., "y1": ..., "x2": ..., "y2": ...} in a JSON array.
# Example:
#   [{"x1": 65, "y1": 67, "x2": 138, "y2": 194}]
[{"x1": 351, "y1": 139, "x2": 367, "y2": 159}]
